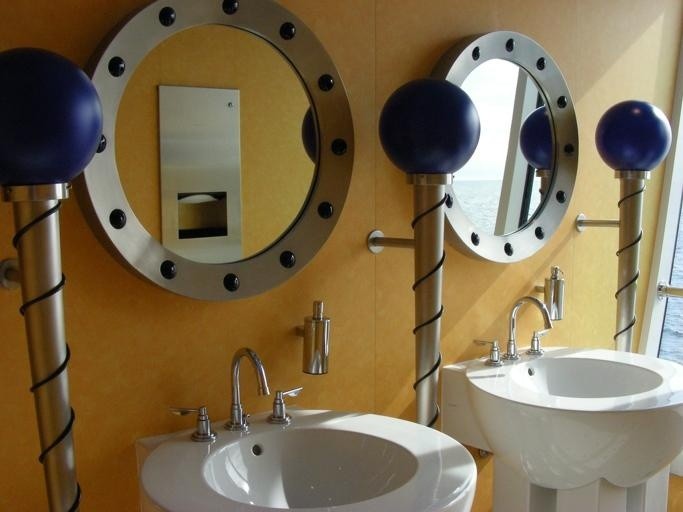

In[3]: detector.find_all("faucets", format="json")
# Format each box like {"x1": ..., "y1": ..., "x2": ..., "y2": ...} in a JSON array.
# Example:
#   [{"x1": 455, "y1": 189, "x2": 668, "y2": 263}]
[
  {"x1": 505, "y1": 295, "x2": 552, "y2": 360},
  {"x1": 225, "y1": 346, "x2": 271, "y2": 430}
]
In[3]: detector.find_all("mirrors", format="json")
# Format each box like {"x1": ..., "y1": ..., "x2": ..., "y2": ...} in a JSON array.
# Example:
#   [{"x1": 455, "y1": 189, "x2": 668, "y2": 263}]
[
  {"x1": 426, "y1": 30, "x2": 578, "y2": 263},
  {"x1": 73, "y1": 0, "x2": 354, "y2": 302}
]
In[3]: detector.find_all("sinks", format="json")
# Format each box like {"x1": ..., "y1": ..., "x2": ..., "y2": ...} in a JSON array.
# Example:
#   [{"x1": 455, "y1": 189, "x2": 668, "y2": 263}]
[
  {"x1": 442, "y1": 346, "x2": 682, "y2": 490},
  {"x1": 135, "y1": 403, "x2": 477, "y2": 511}
]
